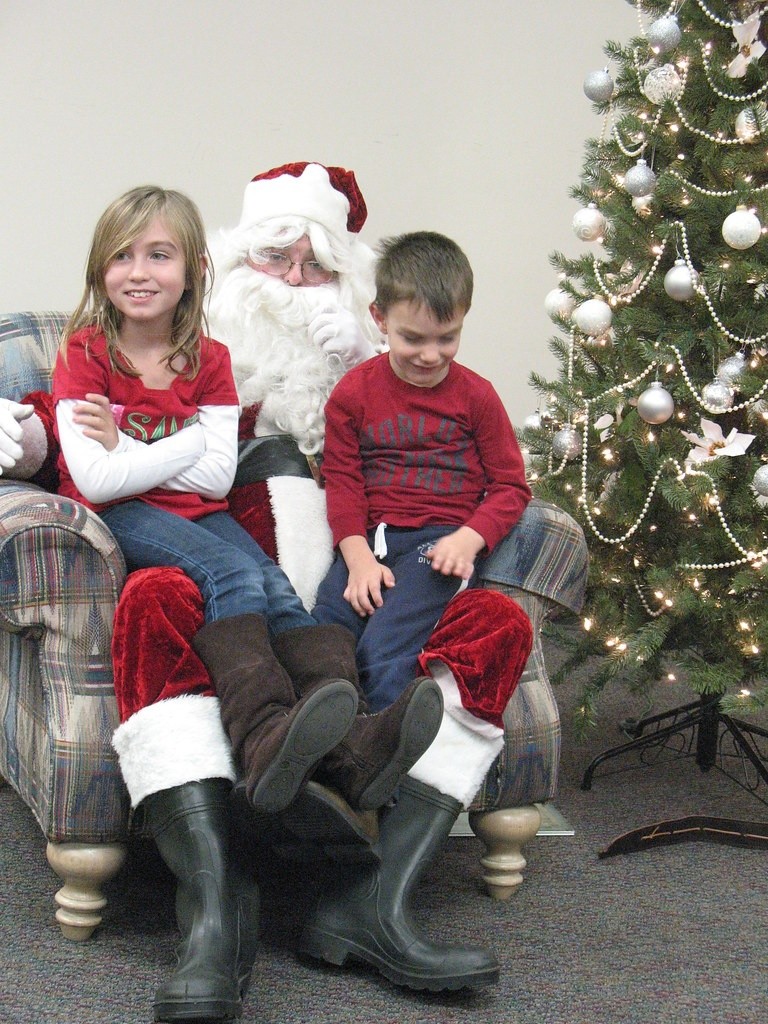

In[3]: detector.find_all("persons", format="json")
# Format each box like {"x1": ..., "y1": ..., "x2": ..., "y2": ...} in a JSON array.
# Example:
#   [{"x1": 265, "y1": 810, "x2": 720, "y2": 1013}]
[
  {"x1": 313, "y1": 230, "x2": 531, "y2": 709},
  {"x1": 0, "y1": 161, "x2": 534, "y2": 1024},
  {"x1": 49, "y1": 187, "x2": 443, "y2": 817}
]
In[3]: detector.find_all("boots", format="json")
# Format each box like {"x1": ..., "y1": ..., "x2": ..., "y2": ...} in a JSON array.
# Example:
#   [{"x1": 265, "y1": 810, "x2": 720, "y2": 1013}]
[
  {"x1": 111, "y1": 694, "x2": 262, "y2": 1023},
  {"x1": 194, "y1": 612, "x2": 360, "y2": 810},
  {"x1": 271, "y1": 623, "x2": 445, "y2": 812},
  {"x1": 291, "y1": 701, "x2": 505, "y2": 993}
]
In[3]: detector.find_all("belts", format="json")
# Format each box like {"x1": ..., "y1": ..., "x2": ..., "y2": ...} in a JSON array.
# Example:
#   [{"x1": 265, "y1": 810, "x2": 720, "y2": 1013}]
[{"x1": 237, "y1": 435, "x2": 326, "y2": 488}]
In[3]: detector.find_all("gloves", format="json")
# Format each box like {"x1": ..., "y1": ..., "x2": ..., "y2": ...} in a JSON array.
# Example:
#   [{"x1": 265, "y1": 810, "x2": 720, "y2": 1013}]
[
  {"x1": 0, "y1": 399, "x2": 34, "y2": 477},
  {"x1": 306, "y1": 303, "x2": 379, "y2": 371}
]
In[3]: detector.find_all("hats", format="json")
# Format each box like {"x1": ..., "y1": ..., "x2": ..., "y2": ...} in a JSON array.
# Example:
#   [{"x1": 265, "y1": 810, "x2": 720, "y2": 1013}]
[{"x1": 239, "y1": 161, "x2": 368, "y2": 249}]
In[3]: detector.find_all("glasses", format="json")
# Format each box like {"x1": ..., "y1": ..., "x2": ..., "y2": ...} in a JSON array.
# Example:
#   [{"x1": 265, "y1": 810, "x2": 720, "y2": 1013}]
[{"x1": 250, "y1": 250, "x2": 333, "y2": 284}]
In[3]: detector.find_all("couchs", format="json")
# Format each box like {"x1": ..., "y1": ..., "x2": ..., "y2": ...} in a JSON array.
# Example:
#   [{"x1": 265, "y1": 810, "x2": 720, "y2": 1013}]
[{"x1": 1, "y1": 313, "x2": 591, "y2": 940}]
